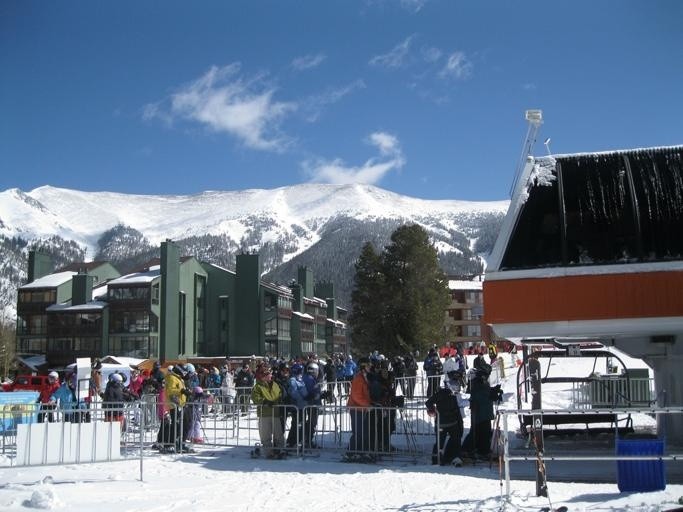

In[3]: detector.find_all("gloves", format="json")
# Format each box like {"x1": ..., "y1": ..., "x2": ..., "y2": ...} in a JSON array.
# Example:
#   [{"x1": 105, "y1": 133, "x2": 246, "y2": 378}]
[{"x1": 181, "y1": 389, "x2": 192, "y2": 395}]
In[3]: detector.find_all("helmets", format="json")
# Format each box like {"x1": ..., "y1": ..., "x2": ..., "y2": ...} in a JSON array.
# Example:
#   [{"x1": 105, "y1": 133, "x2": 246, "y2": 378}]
[
  {"x1": 291, "y1": 364, "x2": 304, "y2": 369},
  {"x1": 376, "y1": 360, "x2": 389, "y2": 370},
  {"x1": 307, "y1": 363, "x2": 318, "y2": 376},
  {"x1": 447, "y1": 371, "x2": 462, "y2": 379},
  {"x1": 173, "y1": 364, "x2": 189, "y2": 376},
  {"x1": 358, "y1": 357, "x2": 371, "y2": 368},
  {"x1": 279, "y1": 362, "x2": 290, "y2": 369}
]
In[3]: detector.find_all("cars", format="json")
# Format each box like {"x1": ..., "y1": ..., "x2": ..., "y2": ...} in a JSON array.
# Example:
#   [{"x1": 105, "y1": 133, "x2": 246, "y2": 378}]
[{"x1": 13, "y1": 375, "x2": 60, "y2": 402}]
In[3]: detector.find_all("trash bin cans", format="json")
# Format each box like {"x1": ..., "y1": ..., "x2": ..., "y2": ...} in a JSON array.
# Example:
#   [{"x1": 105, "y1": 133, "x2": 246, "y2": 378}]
[{"x1": 616, "y1": 439, "x2": 665, "y2": 491}]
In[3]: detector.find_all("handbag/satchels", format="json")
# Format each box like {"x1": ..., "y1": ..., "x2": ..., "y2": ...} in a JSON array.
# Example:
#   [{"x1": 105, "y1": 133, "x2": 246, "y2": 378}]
[
  {"x1": 303, "y1": 394, "x2": 313, "y2": 401},
  {"x1": 434, "y1": 360, "x2": 443, "y2": 371}
]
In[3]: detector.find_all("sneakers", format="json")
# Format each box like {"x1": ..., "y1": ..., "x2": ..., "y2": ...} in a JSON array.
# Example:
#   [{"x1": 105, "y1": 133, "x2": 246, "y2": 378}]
[
  {"x1": 286, "y1": 440, "x2": 320, "y2": 449},
  {"x1": 268, "y1": 449, "x2": 286, "y2": 459},
  {"x1": 344, "y1": 445, "x2": 397, "y2": 461},
  {"x1": 185, "y1": 437, "x2": 202, "y2": 443},
  {"x1": 151, "y1": 442, "x2": 190, "y2": 452}
]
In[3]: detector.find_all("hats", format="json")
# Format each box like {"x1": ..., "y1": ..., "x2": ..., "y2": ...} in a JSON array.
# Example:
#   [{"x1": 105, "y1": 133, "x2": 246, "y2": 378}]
[
  {"x1": 110, "y1": 374, "x2": 122, "y2": 384},
  {"x1": 475, "y1": 363, "x2": 492, "y2": 376},
  {"x1": 186, "y1": 364, "x2": 195, "y2": 372},
  {"x1": 48, "y1": 371, "x2": 59, "y2": 378}
]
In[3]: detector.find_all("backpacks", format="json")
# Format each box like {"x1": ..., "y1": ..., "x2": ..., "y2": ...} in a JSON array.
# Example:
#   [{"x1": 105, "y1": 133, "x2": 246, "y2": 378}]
[{"x1": 425, "y1": 356, "x2": 433, "y2": 370}]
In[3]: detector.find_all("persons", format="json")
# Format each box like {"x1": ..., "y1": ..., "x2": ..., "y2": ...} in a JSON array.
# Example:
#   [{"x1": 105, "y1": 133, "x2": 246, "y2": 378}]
[
  {"x1": 250, "y1": 350, "x2": 358, "y2": 459},
  {"x1": 462, "y1": 340, "x2": 502, "y2": 462},
  {"x1": 48, "y1": 372, "x2": 88, "y2": 423},
  {"x1": 507, "y1": 343, "x2": 518, "y2": 369},
  {"x1": 125, "y1": 363, "x2": 255, "y2": 453},
  {"x1": 99, "y1": 372, "x2": 133, "y2": 423},
  {"x1": 74, "y1": 364, "x2": 96, "y2": 422},
  {"x1": 425, "y1": 371, "x2": 464, "y2": 467},
  {"x1": 344, "y1": 351, "x2": 418, "y2": 463},
  {"x1": 422, "y1": 346, "x2": 469, "y2": 397},
  {"x1": 35, "y1": 372, "x2": 61, "y2": 423}
]
